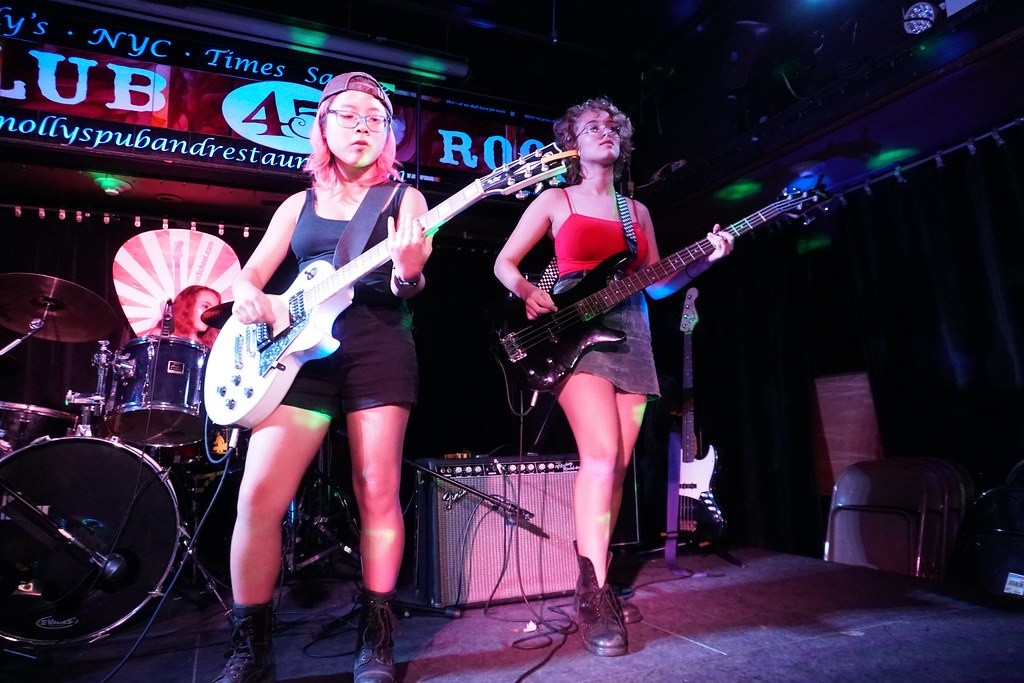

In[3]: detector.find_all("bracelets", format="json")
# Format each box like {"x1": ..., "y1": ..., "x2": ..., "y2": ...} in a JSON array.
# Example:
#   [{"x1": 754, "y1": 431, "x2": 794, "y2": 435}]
[{"x1": 685, "y1": 266, "x2": 696, "y2": 279}]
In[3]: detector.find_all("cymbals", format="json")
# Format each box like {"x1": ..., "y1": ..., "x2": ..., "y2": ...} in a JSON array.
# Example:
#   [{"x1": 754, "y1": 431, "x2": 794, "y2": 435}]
[
  {"x1": 0, "y1": 271, "x2": 120, "y2": 345},
  {"x1": 199, "y1": 301, "x2": 235, "y2": 330}
]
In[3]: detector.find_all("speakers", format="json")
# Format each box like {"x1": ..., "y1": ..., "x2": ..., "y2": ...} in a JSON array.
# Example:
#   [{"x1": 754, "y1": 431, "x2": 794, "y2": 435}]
[
  {"x1": 533, "y1": 389, "x2": 640, "y2": 545},
  {"x1": 395, "y1": 458, "x2": 583, "y2": 609}
]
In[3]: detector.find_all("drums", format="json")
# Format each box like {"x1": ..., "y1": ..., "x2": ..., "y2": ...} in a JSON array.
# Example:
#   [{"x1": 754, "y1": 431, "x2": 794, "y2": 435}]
[
  {"x1": 0, "y1": 435, "x2": 185, "y2": 654},
  {"x1": 103, "y1": 335, "x2": 213, "y2": 449},
  {"x1": 0, "y1": 400, "x2": 84, "y2": 462}
]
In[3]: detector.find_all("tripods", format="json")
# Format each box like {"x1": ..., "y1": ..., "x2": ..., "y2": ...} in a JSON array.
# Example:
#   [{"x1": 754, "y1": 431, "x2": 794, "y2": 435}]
[{"x1": 293, "y1": 426, "x2": 363, "y2": 573}]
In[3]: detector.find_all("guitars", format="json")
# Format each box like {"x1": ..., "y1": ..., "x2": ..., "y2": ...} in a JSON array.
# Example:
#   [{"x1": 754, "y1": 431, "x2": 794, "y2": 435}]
[
  {"x1": 675, "y1": 287, "x2": 731, "y2": 552},
  {"x1": 480, "y1": 183, "x2": 836, "y2": 395},
  {"x1": 203, "y1": 141, "x2": 583, "y2": 430}
]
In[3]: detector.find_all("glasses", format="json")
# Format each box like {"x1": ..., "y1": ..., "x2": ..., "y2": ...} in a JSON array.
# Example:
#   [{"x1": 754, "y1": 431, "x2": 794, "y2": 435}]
[
  {"x1": 573, "y1": 121, "x2": 622, "y2": 140},
  {"x1": 326, "y1": 108, "x2": 390, "y2": 133}
]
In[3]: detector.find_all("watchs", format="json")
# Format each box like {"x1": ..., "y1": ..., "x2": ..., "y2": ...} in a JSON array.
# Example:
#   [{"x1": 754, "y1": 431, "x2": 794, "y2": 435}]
[{"x1": 396, "y1": 272, "x2": 420, "y2": 288}]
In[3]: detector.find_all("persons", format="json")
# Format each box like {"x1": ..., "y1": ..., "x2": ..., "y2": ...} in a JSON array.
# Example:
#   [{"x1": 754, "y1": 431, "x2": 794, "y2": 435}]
[
  {"x1": 215, "y1": 70, "x2": 435, "y2": 683},
  {"x1": 100, "y1": 286, "x2": 223, "y2": 464},
  {"x1": 492, "y1": 98, "x2": 737, "y2": 657}
]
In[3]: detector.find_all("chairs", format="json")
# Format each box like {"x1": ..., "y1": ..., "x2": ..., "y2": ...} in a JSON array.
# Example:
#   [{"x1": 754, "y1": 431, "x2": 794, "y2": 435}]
[{"x1": 821, "y1": 455, "x2": 1024, "y2": 584}]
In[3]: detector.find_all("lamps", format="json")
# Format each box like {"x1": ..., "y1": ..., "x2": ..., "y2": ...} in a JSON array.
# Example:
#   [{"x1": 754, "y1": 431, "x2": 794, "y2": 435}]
[{"x1": 904, "y1": 1, "x2": 942, "y2": 36}]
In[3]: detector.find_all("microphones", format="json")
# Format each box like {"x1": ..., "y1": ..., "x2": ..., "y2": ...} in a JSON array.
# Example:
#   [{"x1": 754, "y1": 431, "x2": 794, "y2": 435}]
[
  {"x1": 97, "y1": 554, "x2": 127, "y2": 581},
  {"x1": 504, "y1": 502, "x2": 533, "y2": 520},
  {"x1": 164, "y1": 300, "x2": 175, "y2": 333}
]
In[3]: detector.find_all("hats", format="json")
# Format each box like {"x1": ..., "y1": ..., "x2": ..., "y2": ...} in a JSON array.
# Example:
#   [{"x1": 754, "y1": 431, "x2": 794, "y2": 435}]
[{"x1": 318, "y1": 72, "x2": 394, "y2": 116}]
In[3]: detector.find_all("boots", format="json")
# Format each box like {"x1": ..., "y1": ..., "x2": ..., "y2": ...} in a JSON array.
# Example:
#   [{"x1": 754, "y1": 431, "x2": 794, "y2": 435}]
[
  {"x1": 210, "y1": 603, "x2": 275, "y2": 683},
  {"x1": 576, "y1": 552, "x2": 628, "y2": 656},
  {"x1": 572, "y1": 540, "x2": 642, "y2": 623},
  {"x1": 352, "y1": 588, "x2": 397, "y2": 683}
]
